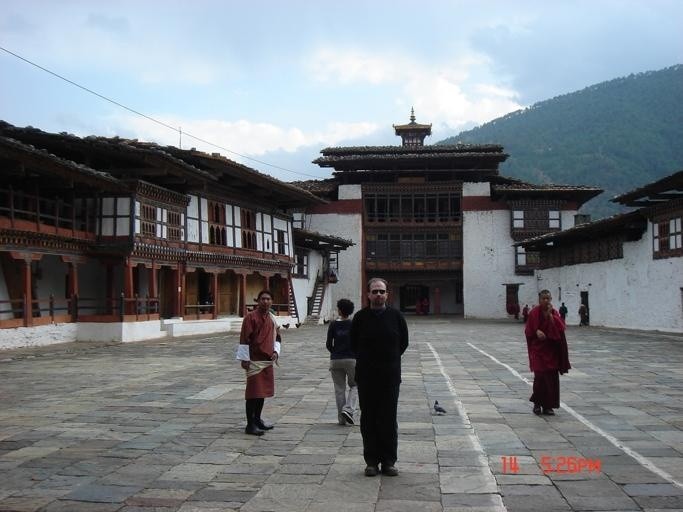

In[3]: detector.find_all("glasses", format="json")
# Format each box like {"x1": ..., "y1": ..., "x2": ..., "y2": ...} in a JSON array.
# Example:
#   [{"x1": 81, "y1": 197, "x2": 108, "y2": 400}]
[{"x1": 370, "y1": 290, "x2": 387, "y2": 294}]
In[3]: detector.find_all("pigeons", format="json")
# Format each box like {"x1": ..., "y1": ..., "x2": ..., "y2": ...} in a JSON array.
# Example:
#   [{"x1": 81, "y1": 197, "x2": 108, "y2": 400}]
[{"x1": 433, "y1": 399, "x2": 446, "y2": 415}]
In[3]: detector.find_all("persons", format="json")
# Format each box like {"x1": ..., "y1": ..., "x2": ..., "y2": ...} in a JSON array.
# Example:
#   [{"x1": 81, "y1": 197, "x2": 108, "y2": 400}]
[
  {"x1": 346, "y1": 276, "x2": 408, "y2": 477},
  {"x1": 523, "y1": 288, "x2": 571, "y2": 417},
  {"x1": 415, "y1": 298, "x2": 421, "y2": 316},
  {"x1": 324, "y1": 297, "x2": 359, "y2": 426},
  {"x1": 234, "y1": 290, "x2": 281, "y2": 437},
  {"x1": 521, "y1": 304, "x2": 530, "y2": 323},
  {"x1": 422, "y1": 297, "x2": 429, "y2": 316},
  {"x1": 512, "y1": 300, "x2": 520, "y2": 320},
  {"x1": 577, "y1": 302, "x2": 587, "y2": 326},
  {"x1": 558, "y1": 302, "x2": 567, "y2": 324}
]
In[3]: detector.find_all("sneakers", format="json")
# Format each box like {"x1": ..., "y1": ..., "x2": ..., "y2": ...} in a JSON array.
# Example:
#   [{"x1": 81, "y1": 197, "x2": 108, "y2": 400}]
[
  {"x1": 543, "y1": 407, "x2": 554, "y2": 415},
  {"x1": 533, "y1": 403, "x2": 541, "y2": 415},
  {"x1": 381, "y1": 464, "x2": 398, "y2": 476},
  {"x1": 338, "y1": 409, "x2": 355, "y2": 425},
  {"x1": 365, "y1": 465, "x2": 379, "y2": 476}
]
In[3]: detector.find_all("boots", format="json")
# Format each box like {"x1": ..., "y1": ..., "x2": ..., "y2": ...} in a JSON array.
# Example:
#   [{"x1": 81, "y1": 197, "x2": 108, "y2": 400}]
[
  {"x1": 255, "y1": 397, "x2": 273, "y2": 430},
  {"x1": 245, "y1": 398, "x2": 265, "y2": 436}
]
